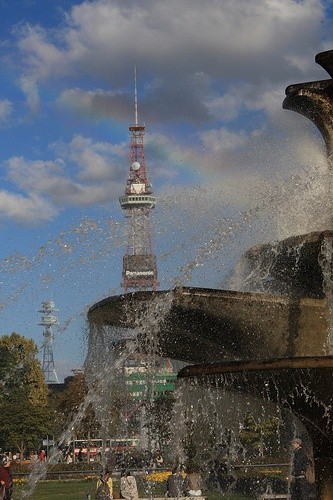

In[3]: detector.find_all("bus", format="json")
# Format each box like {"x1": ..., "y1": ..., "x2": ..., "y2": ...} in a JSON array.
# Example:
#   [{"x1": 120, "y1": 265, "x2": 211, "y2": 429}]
[{"x1": 68, "y1": 439, "x2": 142, "y2": 460}]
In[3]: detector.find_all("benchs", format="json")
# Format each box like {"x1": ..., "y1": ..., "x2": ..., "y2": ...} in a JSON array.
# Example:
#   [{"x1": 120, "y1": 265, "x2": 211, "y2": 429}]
[
  {"x1": 256, "y1": 494, "x2": 293, "y2": 500},
  {"x1": 116, "y1": 496, "x2": 208, "y2": 500}
]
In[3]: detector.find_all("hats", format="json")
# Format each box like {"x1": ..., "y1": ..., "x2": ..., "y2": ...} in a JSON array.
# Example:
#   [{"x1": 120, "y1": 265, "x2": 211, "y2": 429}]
[{"x1": 290, "y1": 438, "x2": 302, "y2": 443}]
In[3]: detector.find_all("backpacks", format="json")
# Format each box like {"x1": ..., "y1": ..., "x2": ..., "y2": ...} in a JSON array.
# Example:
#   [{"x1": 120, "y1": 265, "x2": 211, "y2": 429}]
[{"x1": 96, "y1": 478, "x2": 110, "y2": 500}]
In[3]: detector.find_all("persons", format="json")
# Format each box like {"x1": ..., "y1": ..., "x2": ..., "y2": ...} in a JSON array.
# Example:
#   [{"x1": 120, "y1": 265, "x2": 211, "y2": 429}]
[
  {"x1": 291, "y1": 438, "x2": 308, "y2": 500},
  {"x1": 94, "y1": 471, "x2": 113, "y2": 500},
  {"x1": 155, "y1": 451, "x2": 163, "y2": 469},
  {"x1": 78, "y1": 448, "x2": 82, "y2": 460},
  {"x1": 184, "y1": 471, "x2": 190, "y2": 483},
  {"x1": 63, "y1": 448, "x2": 70, "y2": 460},
  {"x1": 185, "y1": 469, "x2": 202, "y2": 495},
  {"x1": 41, "y1": 448, "x2": 45, "y2": 462},
  {"x1": 120, "y1": 469, "x2": 138, "y2": 500},
  {"x1": 167, "y1": 467, "x2": 185, "y2": 498},
  {"x1": 0, "y1": 459, "x2": 13, "y2": 500}
]
